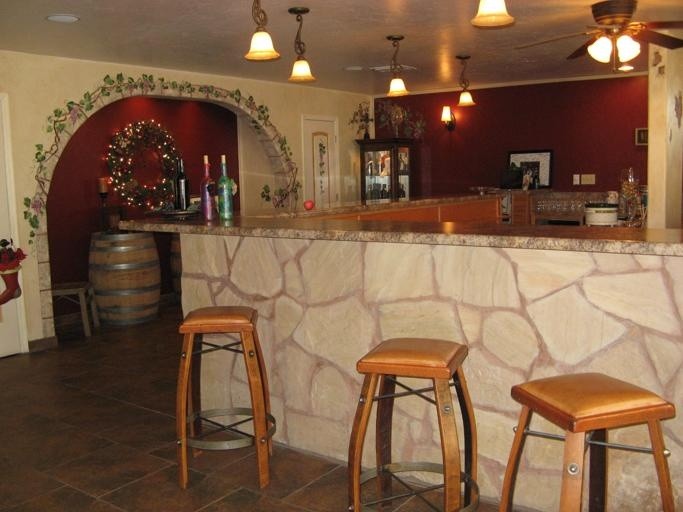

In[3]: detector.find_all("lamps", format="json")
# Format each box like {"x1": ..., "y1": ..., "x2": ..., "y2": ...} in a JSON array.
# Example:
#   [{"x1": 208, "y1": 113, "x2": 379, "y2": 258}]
[
  {"x1": 470, "y1": 0, "x2": 515, "y2": 29},
  {"x1": 617, "y1": 64, "x2": 634, "y2": 72},
  {"x1": 386, "y1": 35, "x2": 410, "y2": 97},
  {"x1": 288, "y1": 6, "x2": 316, "y2": 81},
  {"x1": 587, "y1": 34, "x2": 641, "y2": 63},
  {"x1": 244, "y1": 0, "x2": 280, "y2": 61},
  {"x1": 441, "y1": 106, "x2": 452, "y2": 127},
  {"x1": 455, "y1": 55, "x2": 477, "y2": 107}
]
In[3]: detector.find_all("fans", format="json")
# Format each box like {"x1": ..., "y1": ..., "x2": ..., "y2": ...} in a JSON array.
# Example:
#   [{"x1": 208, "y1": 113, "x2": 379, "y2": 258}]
[{"x1": 513, "y1": 0, "x2": 680, "y2": 60}]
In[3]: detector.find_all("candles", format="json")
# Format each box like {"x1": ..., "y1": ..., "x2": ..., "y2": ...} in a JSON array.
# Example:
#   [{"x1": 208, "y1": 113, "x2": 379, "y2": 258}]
[{"x1": 96, "y1": 178, "x2": 108, "y2": 192}]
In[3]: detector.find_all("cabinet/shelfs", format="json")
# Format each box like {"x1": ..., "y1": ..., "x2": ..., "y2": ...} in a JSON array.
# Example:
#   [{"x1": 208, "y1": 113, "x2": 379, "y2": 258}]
[{"x1": 355, "y1": 139, "x2": 421, "y2": 205}]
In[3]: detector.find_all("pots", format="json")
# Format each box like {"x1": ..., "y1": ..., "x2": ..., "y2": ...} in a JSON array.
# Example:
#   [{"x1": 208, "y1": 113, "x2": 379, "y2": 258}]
[{"x1": 584, "y1": 201, "x2": 619, "y2": 229}]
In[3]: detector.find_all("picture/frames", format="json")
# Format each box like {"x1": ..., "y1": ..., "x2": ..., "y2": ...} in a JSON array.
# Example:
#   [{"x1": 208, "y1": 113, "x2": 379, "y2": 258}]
[
  {"x1": 506, "y1": 149, "x2": 554, "y2": 188},
  {"x1": 634, "y1": 127, "x2": 648, "y2": 146}
]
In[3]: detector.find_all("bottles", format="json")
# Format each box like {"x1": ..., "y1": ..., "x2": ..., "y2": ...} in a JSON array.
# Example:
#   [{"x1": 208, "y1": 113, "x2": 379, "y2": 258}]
[
  {"x1": 174, "y1": 158, "x2": 190, "y2": 211},
  {"x1": 366, "y1": 185, "x2": 372, "y2": 199},
  {"x1": 635, "y1": 176, "x2": 642, "y2": 216},
  {"x1": 533, "y1": 166, "x2": 540, "y2": 189},
  {"x1": 403, "y1": 153, "x2": 409, "y2": 171},
  {"x1": 618, "y1": 170, "x2": 628, "y2": 219},
  {"x1": 381, "y1": 184, "x2": 388, "y2": 198},
  {"x1": 216, "y1": 155, "x2": 234, "y2": 219},
  {"x1": 201, "y1": 155, "x2": 219, "y2": 221},
  {"x1": 627, "y1": 167, "x2": 637, "y2": 217}
]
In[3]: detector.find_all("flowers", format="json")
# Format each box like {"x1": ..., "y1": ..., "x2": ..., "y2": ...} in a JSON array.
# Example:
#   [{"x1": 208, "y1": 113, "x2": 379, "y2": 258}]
[
  {"x1": 375, "y1": 98, "x2": 403, "y2": 128},
  {"x1": 23, "y1": 72, "x2": 303, "y2": 245},
  {"x1": 104, "y1": 119, "x2": 191, "y2": 211},
  {"x1": 347, "y1": 99, "x2": 374, "y2": 134}
]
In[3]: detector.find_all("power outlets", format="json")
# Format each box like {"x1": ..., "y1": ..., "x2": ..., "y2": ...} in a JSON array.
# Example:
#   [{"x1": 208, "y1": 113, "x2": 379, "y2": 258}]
[{"x1": 581, "y1": 173, "x2": 596, "y2": 185}]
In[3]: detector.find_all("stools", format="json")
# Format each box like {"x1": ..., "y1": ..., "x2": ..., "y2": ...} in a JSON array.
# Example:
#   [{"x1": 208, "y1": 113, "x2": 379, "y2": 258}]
[
  {"x1": 498, "y1": 372, "x2": 676, "y2": 512},
  {"x1": 347, "y1": 337, "x2": 480, "y2": 510},
  {"x1": 52, "y1": 281, "x2": 102, "y2": 337},
  {"x1": 175, "y1": 305, "x2": 277, "y2": 490}
]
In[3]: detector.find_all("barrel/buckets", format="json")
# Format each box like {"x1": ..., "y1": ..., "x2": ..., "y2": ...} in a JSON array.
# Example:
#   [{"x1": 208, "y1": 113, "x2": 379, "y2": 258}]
[
  {"x1": 167, "y1": 231, "x2": 183, "y2": 312},
  {"x1": 87, "y1": 226, "x2": 163, "y2": 329}
]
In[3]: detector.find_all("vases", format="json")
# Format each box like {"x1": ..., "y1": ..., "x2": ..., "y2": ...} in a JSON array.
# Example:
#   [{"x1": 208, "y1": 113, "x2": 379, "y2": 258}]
[
  {"x1": 394, "y1": 124, "x2": 400, "y2": 138},
  {"x1": 364, "y1": 128, "x2": 370, "y2": 140}
]
